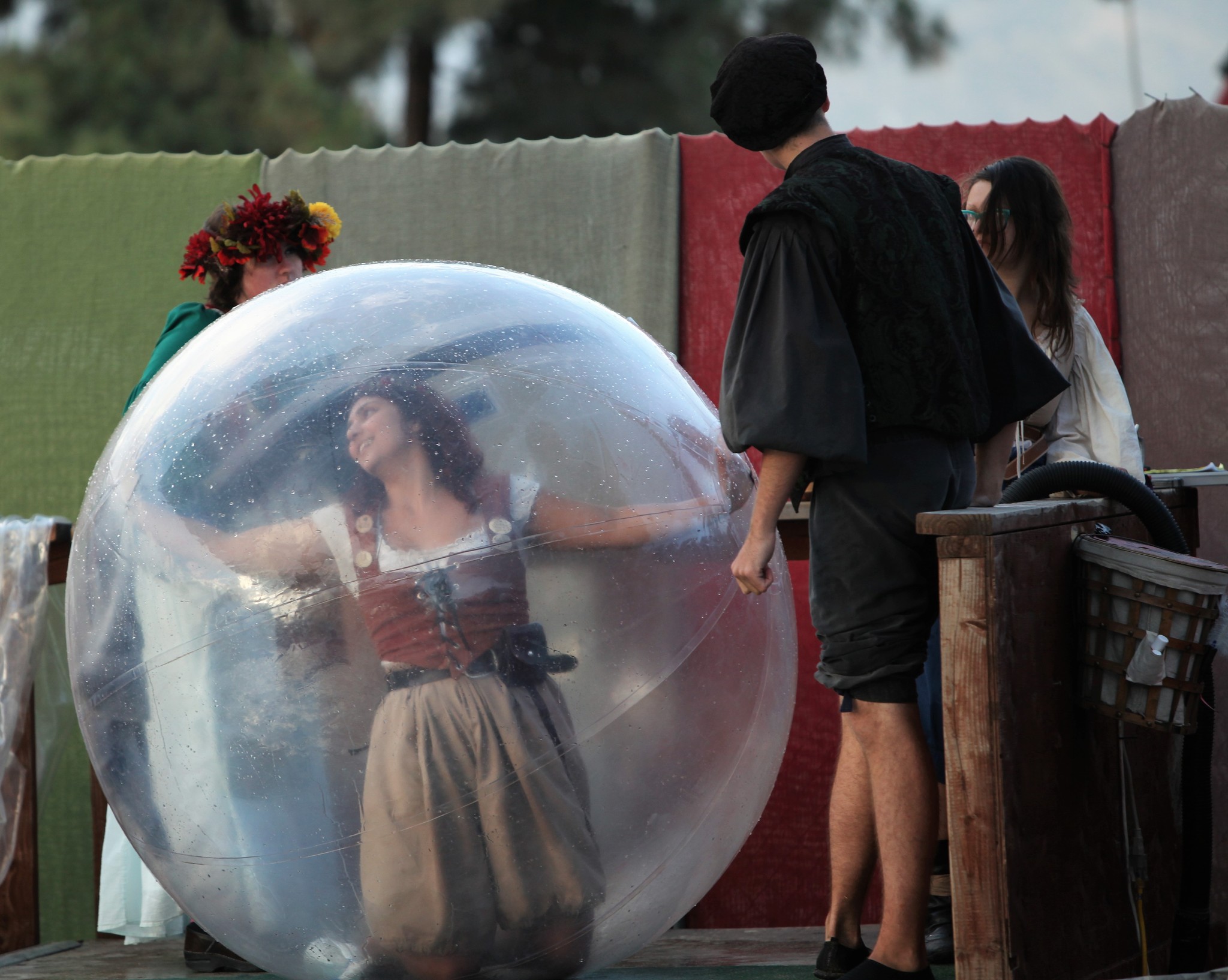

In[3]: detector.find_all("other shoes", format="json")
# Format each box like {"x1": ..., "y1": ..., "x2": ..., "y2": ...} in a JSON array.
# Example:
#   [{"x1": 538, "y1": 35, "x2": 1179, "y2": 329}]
[
  {"x1": 813, "y1": 943, "x2": 873, "y2": 977},
  {"x1": 840, "y1": 954, "x2": 934, "y2": 980}
]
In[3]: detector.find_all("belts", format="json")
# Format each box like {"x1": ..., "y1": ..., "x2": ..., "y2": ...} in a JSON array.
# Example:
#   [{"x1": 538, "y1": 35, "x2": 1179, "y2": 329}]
[{"x1": 387, "y1": 650, "x2": 578, "y2": 690}]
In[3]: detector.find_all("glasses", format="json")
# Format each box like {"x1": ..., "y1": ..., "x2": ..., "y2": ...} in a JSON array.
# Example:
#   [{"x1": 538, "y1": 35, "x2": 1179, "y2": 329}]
[{"x1": 962, "y1": 209, "x2": 1010, "y2": 232}]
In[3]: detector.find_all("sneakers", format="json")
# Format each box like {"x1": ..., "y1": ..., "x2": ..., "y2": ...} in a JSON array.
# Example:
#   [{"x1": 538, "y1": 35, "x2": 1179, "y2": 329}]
[{"x1": 924, "y1": 915, "x2": 954, "y2": 953}]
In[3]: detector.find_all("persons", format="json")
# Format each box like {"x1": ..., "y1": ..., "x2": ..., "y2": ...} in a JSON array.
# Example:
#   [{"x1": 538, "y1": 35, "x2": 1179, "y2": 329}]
[
  {"x1": 889, "y1": 160, "x2": 1147, "y2": 958},
  {"x1": 97, "y1": 189, "x2": 748, "y2": 980},
  {"x1": 713, "y1": 36, "x2": 1075, "y2": 980}
]
